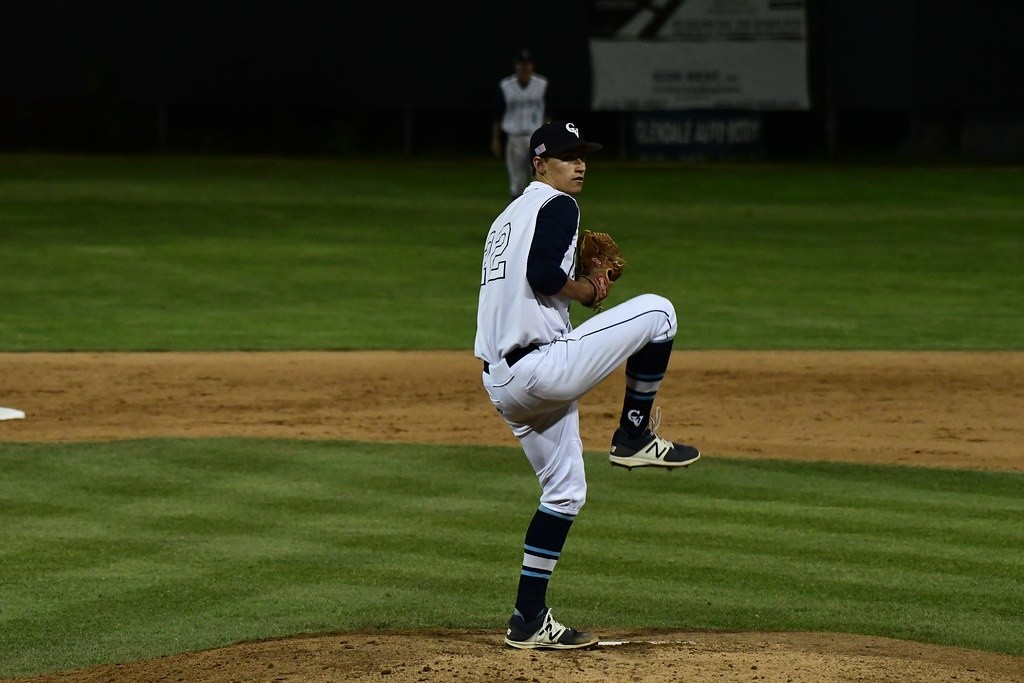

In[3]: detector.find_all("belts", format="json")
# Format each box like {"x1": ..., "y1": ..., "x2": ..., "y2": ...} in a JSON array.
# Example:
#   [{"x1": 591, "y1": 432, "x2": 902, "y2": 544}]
[{"x1": 484, "y1": 341, "x2": 537, "y2": 374}]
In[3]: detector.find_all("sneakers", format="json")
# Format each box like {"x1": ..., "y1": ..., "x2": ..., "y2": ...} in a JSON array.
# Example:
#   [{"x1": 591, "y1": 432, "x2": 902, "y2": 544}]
[
  {"x1": 505, "y1": 607, "x2": 598, "y2": 650},
  {"x1": 608, "y1": 407, "x2": 701, "y2": 472}
]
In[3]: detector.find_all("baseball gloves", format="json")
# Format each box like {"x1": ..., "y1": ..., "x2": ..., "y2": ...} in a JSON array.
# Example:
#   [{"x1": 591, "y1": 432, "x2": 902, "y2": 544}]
[{"x1": 574, "y1": 228, "x2": 627, "y2": 309}]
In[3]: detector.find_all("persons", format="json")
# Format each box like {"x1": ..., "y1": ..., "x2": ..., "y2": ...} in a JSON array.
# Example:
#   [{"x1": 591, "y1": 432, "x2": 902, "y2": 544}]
[
  {"x1": 472, "y1": 121, "x2": 700, "y2": 650},
  {"x1": 490, "y1": 48, "x2": 553, "y2": 197}
]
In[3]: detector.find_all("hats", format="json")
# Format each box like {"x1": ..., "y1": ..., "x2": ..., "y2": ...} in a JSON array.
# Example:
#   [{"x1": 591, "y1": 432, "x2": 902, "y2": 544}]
[
  {"x1": 515, "y1": 48, "x2": 532, "y2": 63},
  {"x1": 530, "y1": 121, "x2": 602, "y2": 167}
]
